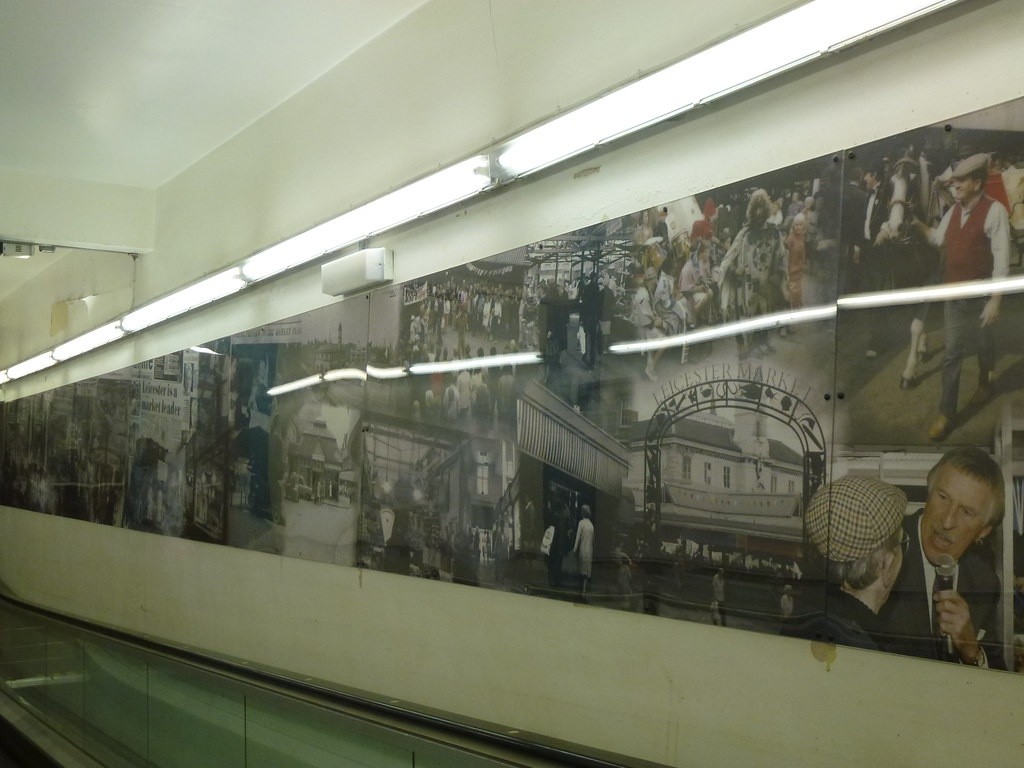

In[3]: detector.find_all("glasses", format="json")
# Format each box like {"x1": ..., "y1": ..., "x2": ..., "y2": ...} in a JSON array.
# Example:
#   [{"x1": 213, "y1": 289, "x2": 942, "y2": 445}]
[{"x1": 884, "y1": 530, "x2": 910, "y2": 557}]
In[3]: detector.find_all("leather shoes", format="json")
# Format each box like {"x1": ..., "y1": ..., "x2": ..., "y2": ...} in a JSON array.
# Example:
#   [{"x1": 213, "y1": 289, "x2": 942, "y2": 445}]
[
  {"x1": 971, "y1": 383, "x2": 996, "y2": 406},
  {"x1": 927, "y1": 415, "x2": 954, "y2": 438}
]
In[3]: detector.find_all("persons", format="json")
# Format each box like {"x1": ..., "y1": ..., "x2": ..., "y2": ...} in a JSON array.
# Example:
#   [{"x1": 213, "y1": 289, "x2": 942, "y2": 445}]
[
  {"x1": 534, "y1": 496, "x2": 796, "y2": 632},
  {"x1": 776, "y1": 475, "x2": 907, "y2": 650},
  {"x1": 314, "y1": 479, "x2": 356, "y2": 505},
  {"x1": 868, "y1": 444, "x2": 1007, "y2": 673},
  {"x1": 379, "y1": 504, "x2": 510, "y2": 587},
  {"x1": 630, "y1": 184, "x2": 838, "y2": 380},
  {"x1": 398, "y1": 254, "x2": 629, "y2": 436},
  {"x1": 233, "y1": 355, "x2": 269, "y2": 415},
  {"x1": 840, "y1": 113, "x2": 1024, "y2": 441}
]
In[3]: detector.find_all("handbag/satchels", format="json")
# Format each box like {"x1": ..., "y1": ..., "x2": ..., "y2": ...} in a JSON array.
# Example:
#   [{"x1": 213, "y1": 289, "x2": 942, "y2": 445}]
[
  {"x1": 541, "y1": 524, "x2": 554, "y2": 555},
  {"x1": 710, "y1": 598, "x2": 723, "y2": 626},
  {"x1": 561, "y1": 549, "x2": 578, "y2": 574}
]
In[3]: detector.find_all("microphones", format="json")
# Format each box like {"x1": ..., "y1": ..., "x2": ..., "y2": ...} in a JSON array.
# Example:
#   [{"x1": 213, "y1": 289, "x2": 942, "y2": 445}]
[{"x1": 934, "y1": 554, "x2": 957, "y2": 654}]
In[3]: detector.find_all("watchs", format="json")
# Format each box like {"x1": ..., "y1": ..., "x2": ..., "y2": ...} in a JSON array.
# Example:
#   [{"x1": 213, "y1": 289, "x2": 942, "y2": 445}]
[{"x1": 968, "y1": 647, "x2": 985, "y2": 667}]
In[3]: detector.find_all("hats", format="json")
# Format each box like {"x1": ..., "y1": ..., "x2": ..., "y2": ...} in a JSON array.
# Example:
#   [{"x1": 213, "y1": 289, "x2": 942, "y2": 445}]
[
  {"x1": 804, "y1": 474, "x2": 907, "y2": 562},
  {"x1": 951, "y1": 153, "x2": 989, "y2": 179}
]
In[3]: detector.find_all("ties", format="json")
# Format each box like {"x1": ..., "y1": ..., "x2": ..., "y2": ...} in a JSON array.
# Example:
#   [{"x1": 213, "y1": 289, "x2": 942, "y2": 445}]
[{"x1": 931, "y1": 568, "x2": 950, "y2": 663}]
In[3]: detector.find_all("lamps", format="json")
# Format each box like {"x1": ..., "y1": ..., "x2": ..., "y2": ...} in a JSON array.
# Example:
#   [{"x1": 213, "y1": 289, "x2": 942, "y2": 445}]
[{"x1": 1, "y1": 0, "x2": 1024, "y2": 386}]
